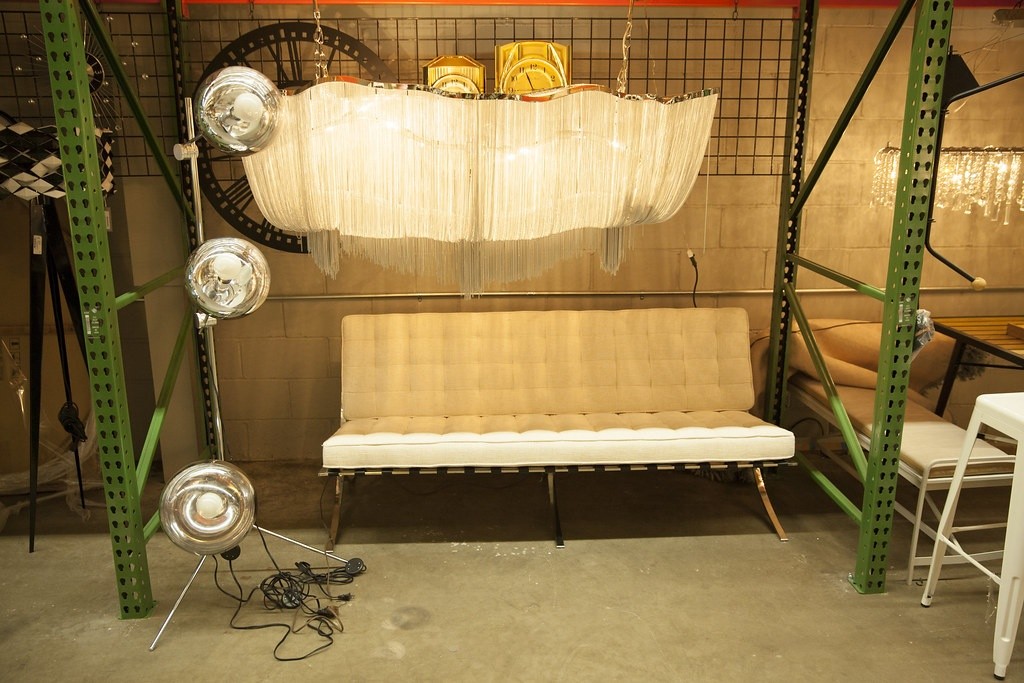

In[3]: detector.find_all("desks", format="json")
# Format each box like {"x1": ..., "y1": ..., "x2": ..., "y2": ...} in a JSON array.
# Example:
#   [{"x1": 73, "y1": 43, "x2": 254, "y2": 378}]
[{"x1": 929, "y1": 315, "x2": 1024, "y2": 418}]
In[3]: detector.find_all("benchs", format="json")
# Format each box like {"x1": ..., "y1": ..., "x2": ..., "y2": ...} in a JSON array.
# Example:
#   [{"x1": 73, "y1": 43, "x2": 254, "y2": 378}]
[
  {"x1": 787, "y1": 371, "x2": 1015, "y2": 586},
  {"x1": 316, "y1": 307, "x2": 798, "y2": 553}
]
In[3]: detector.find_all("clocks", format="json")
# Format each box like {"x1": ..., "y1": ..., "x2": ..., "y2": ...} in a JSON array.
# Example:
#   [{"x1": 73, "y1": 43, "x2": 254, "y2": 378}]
[
  {"x1": 422, "y1": 54, "x2": 487, "y2": 93},
  {"x1": 21, "y1": 18, "x2": 126, "y2": 129},
  {"x1": 494, "y1": 40, "x2": 572, "y2": 94}
]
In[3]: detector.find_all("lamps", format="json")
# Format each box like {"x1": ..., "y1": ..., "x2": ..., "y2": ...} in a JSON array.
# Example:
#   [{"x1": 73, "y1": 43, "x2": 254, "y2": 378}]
[
  {"x1": 241, "y1": 0, "x2": 721, "y2": 302},
  {"x1": 869, "y1": 71, "x2": 1024, "y2": 225}
]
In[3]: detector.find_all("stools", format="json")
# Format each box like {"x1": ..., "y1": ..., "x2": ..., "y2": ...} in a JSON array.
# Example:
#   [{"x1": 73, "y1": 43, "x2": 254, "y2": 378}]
[{"x1": 920, "y1": 391, "x2": 1024, "y2": 681}]
